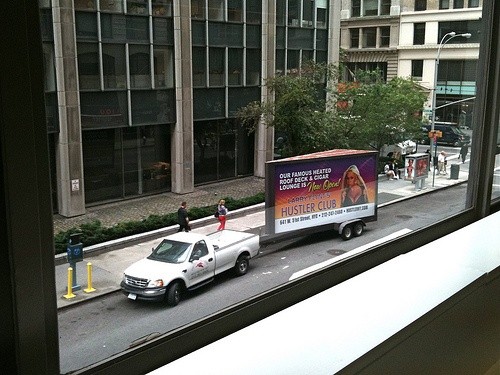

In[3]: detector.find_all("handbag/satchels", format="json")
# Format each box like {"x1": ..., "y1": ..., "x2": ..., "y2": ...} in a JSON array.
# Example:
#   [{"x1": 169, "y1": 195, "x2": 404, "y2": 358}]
[{"x1": 215, "y1": 211, "x2": 219, "y2": 218}]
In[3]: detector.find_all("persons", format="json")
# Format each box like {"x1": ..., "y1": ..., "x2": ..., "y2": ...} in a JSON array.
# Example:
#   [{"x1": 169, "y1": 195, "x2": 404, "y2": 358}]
[
  {"x1": 436, "y1": 143, "x2": 468, "y2": 175},
  {"x1": 384, "y1": 161, "x2": 403, "y2": 181},
  {"x1": 425, "y1": 149, "x2": 432, "y2": 171},
  {"x1": 405, "y1": 159, "x2": 427, "y2": 180},
  {"x1": 341, "y1": 165, "x2": 369, "y2": 208},
  {"x1": 176, "y1": 202, "x2": 189, "y2": 232},
  {"x1": 217, "y1": 199, "x2": 227, "y2": 231}
]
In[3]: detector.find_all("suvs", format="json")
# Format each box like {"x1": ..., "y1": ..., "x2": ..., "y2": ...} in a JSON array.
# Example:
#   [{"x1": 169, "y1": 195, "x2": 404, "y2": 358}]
[{"x1": 413, "y1": 122, "x2": 471, "y2": 147}]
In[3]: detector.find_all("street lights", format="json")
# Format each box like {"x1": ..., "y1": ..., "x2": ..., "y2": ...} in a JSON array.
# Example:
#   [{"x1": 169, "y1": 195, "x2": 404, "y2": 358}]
[{"x1": 429, "y1": 31, "x2": 471, "y2": 168}]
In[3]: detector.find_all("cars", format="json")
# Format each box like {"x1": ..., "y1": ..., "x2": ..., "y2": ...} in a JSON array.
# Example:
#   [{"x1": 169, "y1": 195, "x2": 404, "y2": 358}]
[{"x1": 368, "y1": 130, "x2": 416, "y2": 158}]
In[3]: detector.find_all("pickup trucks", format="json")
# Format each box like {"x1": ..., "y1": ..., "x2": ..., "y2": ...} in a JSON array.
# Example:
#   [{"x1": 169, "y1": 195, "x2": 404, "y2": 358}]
[{"x1": 120, "y1": 228, "x2": 261, "y2": 307}]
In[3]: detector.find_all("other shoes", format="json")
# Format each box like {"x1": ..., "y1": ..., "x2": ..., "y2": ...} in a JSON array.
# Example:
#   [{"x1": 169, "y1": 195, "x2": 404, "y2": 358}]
[
  {"x1": 400, "y1": 178, "x2": 402, "y2": 180},
  {"x1": 389, "y1": 178, "x2": 393, "y2": 181}
]
